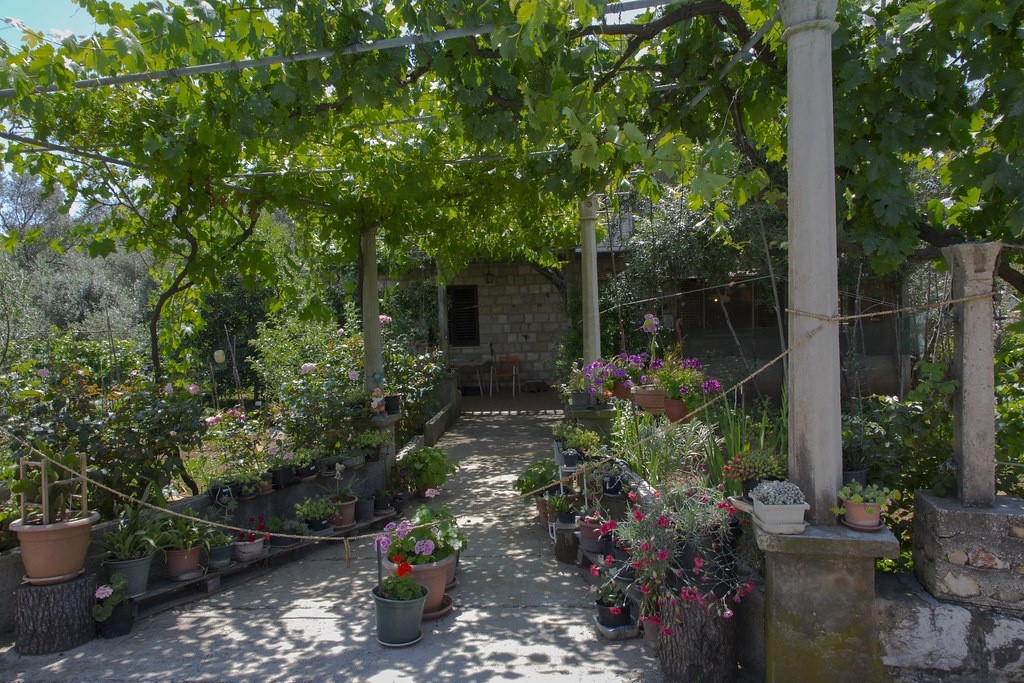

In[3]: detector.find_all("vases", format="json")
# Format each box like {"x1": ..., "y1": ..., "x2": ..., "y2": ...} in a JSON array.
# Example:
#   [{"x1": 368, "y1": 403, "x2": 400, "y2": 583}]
[
  {"x1": 446, "y1": 551, "x2": 459, "y2": 589},
  {"x1": 371, "y1": 585, "x2": 430, "y2": 646},
  {"x1": 571, "y1": 378, "x2": 692, "y2": 647},
  {"x1": 382, "y1": 556, "x2": 455, "y2": 620},
  {"x1": 668, "y1": 519, "x2": 738, "y2": 595}
]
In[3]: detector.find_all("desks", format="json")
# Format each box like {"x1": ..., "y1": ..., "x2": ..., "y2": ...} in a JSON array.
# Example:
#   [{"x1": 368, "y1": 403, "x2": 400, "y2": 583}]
[{"x1": 451, "y1": 359, "x2": 489, "y2": 396}]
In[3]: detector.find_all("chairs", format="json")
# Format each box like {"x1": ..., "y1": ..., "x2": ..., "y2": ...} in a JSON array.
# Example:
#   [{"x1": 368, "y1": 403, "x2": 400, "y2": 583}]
[{"x1": 489, "y1": 356, "x2": 521, "y2": 397}]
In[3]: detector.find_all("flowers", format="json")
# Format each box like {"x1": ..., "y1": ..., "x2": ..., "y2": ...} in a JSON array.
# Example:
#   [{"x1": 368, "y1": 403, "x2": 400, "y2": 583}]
[
  {"x1": 374, "y1": 521, "x2": 449, "y2": 564},
  {"x1": 95, "y1": 572, "x2": 131, "y2": 621},
  {"x1": 412, "y1": 490, "x2": 469, "y2": 552},
  {"x1": 231, "y1": 513, "x2": 270, "y2": 542},
  {"x1": 585, "y1": 470, "x2": 757, "y2": 648},
  {"x1": 376, "y1": 554, "x2": 422, "y2": 600},
  {"x1": 574, "y1": 313, "x2": 723, "y2": 397},
  {"x1": 11, "y1": 315, "x2": 443, "y2": 436}
]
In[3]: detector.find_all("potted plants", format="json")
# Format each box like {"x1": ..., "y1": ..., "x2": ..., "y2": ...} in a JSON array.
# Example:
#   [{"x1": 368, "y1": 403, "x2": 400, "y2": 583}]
[
  {"x1": 724, "y1": 445, "x2": 901, "y2": 533},
  {"x1": 99, "y1": 598, "x2": 134, "y2": 638},
  {"x1": 231, "y1": 536, "x2": 265, "y2": 562},
  {"x1": 515, "y1": 424, "x2": 623, "y2": 564},
  {"x1": 9, "y1": 397, "x2": 459, "y2": 647}
]
